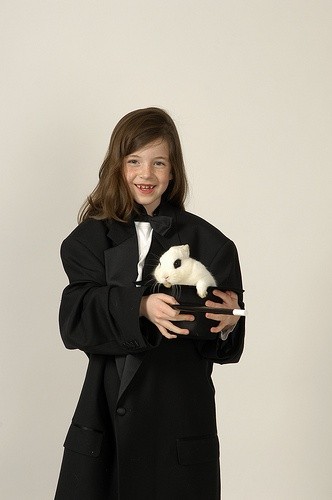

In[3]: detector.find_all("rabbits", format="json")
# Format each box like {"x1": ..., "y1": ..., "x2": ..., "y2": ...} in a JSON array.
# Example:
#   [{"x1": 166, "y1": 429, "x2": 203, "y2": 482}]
[{"x1": 142, "y1": 244, "x2": 218, "y2": 300}]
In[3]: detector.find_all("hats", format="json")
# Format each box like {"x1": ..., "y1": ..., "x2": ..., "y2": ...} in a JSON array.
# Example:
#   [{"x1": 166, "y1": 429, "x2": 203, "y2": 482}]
[{"x1": 133, "y1": 280, "x2": 244, "y2": 340}]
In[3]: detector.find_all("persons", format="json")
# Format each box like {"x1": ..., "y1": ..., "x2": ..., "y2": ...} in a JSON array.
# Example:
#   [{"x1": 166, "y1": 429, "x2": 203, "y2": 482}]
[{"x1": 54, "y1": 107, "x2": 245, "y2": 500}]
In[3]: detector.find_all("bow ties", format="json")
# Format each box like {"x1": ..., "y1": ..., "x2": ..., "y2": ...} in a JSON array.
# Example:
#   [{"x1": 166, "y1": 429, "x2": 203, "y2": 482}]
[{"x1": 132, "y1": 203, "x2": 173, "y2": 237}]
